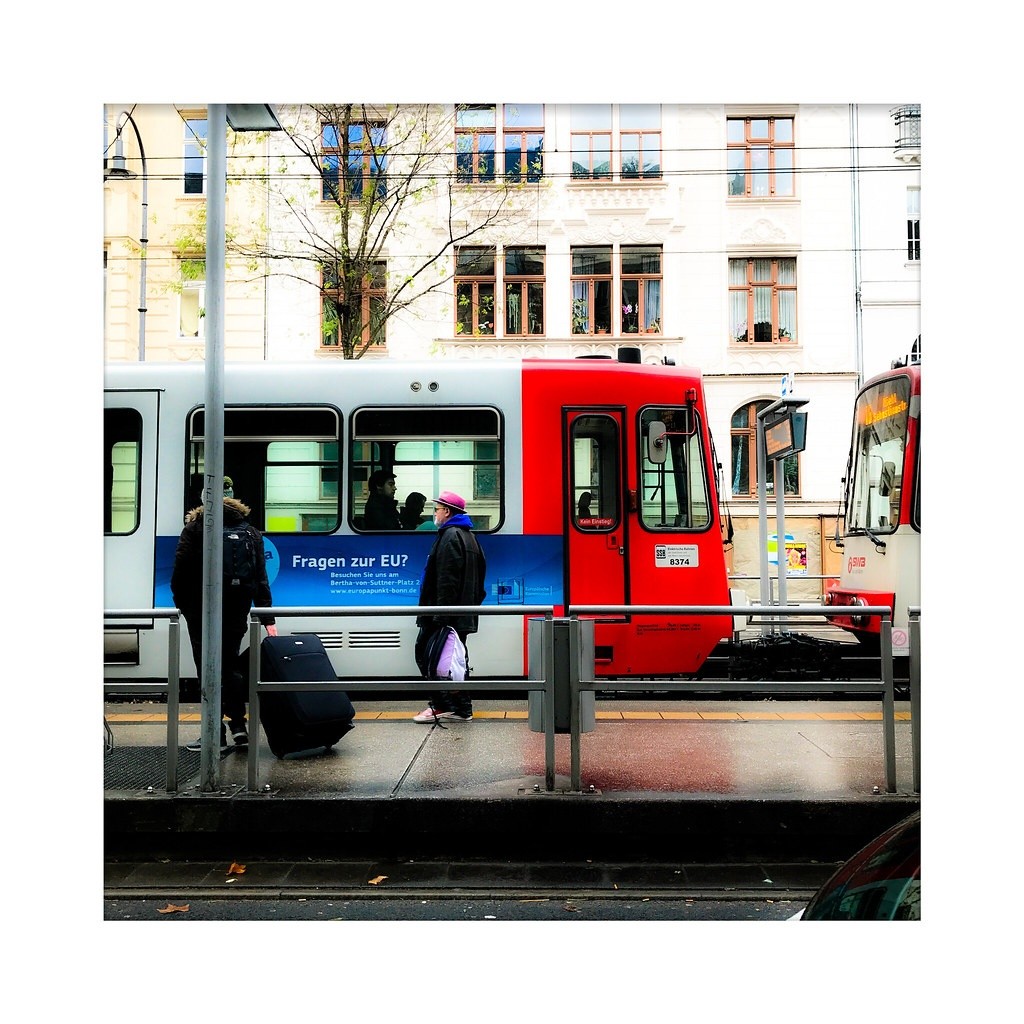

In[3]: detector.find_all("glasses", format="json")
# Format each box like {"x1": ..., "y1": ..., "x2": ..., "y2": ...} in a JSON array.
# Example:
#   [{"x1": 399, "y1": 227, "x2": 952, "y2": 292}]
[{"x1": 433, "y1": 506, "x2": 445, "y2": 511}]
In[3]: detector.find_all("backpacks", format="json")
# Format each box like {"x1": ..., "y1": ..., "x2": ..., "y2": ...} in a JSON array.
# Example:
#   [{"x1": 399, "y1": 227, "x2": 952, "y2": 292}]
[{"x1": 425, "y1": 624, "x2": 466, "y2": 691}]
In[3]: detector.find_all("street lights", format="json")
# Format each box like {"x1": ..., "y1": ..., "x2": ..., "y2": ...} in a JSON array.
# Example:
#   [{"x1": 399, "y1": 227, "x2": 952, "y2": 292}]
[{"x1": 198, "y1": 103, "x2": 285, "y2": 789}]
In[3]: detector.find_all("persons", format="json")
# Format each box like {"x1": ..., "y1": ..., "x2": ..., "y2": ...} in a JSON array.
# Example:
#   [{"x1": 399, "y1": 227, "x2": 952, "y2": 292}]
[
  {"x1": 398, "y1": 489, "x2": 428, "y2": 529},
  {"x1": 411, "y1": 490, "x2": 489, "y2": 723},
  {"x1": 577, "y1": 491, "x2": 593, "y2": 519},
  {"x1": 362, "y1": 471, "x2": 403, "y2": 532},
  {"x1": 167, "y1": 475, "x2": 278, "y2": 752}
]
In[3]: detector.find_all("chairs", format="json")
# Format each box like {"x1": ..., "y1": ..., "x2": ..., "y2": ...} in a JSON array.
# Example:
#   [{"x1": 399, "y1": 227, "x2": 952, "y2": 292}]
[{"x1": 578, "y1": 492, "x2": 599, "y2": 531}]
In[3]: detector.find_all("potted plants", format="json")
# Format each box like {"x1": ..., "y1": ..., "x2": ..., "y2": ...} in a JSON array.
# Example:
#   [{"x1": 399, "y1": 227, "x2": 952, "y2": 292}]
[
  {"x1": 647, "y1": 324, "x2": 655, "y2": 333},
  {"x1": 596, "y1": 325, "x2": 607, "y2": 334},
  {"x1": 779, "y1": 328, "x2": 791, "y2": 343}
]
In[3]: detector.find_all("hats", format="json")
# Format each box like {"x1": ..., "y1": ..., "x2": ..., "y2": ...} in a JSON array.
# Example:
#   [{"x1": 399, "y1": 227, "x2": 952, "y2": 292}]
[
  {"x1": 432, "y1": 491, "x2": 468, "y2": 512},
  {"x1": 202, "y1": 476, "x2": 233, "y2": 505}
]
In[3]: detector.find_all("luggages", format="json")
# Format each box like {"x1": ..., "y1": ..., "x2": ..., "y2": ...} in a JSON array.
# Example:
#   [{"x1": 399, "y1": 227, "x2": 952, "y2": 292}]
[{"x1": 239, "y1": 633, "x2": 354, "y2": 762}]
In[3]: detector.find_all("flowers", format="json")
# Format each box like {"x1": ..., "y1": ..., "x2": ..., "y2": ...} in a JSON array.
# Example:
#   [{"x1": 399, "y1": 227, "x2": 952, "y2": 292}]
[{"x1": 623, "y1": 302, "x2": 639, "y2": 332}]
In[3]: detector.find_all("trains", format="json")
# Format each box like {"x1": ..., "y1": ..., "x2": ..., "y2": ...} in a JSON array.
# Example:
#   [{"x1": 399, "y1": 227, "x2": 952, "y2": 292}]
[{"x1": 103, "y1": 343, "x2": 920, "y2": 691}]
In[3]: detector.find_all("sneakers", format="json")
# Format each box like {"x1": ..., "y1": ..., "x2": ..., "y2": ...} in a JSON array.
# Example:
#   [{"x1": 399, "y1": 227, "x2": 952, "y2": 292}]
[
  {"x1": 232, "y1": 724, "x2": 249, "y2": 745},
  {"x1": 412, "y1": 707, "x2": 453, "y2": 720},
  {"x1": 446, "y1": 711, "x2": 471, "y2": 721},
  {"x1": 187, "y1": 736, "x2": 229, "y2": 752}
]
